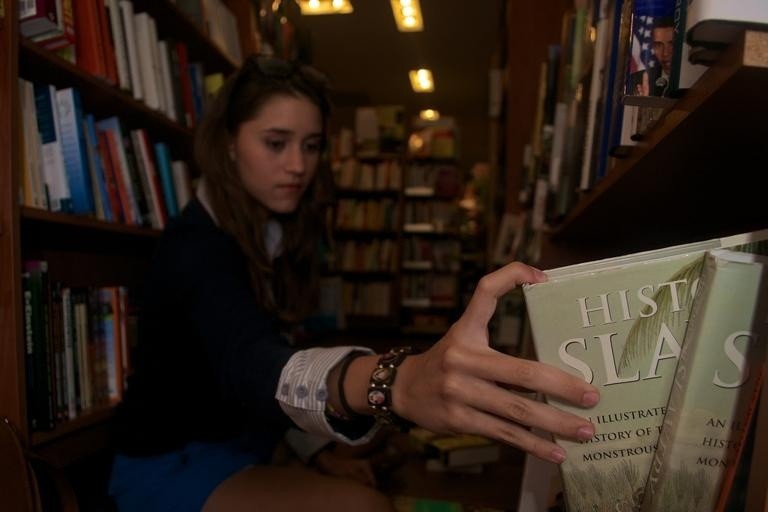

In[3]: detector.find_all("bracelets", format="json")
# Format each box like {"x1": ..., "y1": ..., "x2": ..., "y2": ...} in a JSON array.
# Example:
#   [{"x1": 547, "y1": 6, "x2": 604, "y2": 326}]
[
  {"x1": 367, "y1": 347, "x2": 414, "y2": 427},
  {"x1": 337, "y1": 352, "x2": 366, "y2": 419}
]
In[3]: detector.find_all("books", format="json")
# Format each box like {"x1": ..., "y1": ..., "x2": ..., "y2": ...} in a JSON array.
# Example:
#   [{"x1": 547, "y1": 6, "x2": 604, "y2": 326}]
[
  {"x1": 520, "y1": 228, "x2": 767, "y2": 511},
  {"x1": 18, "y1": 2, "x2": 224, "y2": 437},
  {"x1": 308, "y1": 106, "x2": 497, "y2": 332},
  {"x1": 516, "y1": 2, "x2": 767, "y2": 231}
]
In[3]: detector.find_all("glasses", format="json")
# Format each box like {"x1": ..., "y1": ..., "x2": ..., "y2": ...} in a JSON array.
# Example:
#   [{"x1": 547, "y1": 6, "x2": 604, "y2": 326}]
[{"x1": 246, "y1": 54, "x2": 331, "y2": 96}]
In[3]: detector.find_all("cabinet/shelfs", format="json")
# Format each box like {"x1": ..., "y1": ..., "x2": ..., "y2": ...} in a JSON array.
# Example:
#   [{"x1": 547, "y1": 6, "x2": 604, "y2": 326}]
[
  {"x1": 489, "y1": 3, "x2": 765, "y2": 510},
  {"x1": 304, "y1": 100, "x2": 486, "y2": 368},
  {"x1": 2, "y1": 1, "x2": 314, "y2": 509}
]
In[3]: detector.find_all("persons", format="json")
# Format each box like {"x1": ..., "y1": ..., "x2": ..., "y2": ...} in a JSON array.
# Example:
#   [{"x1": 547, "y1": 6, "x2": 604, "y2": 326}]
[{"x1": 105, "y1": 51, "x2": 600, "y2": 511}]
